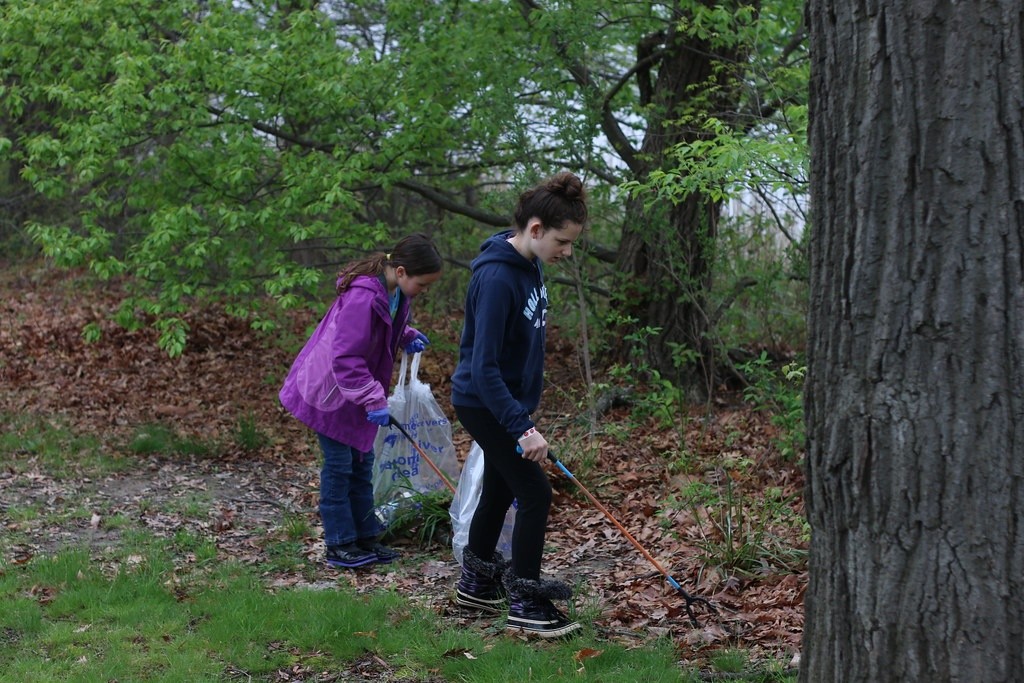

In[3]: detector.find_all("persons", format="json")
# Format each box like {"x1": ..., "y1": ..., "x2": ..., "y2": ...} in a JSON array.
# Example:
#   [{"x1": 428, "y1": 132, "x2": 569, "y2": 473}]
[
  {"x1": 278, "y1": 233, "x2": 445, "y2": 568},
  {"x1": 451, "y1": 171, "x2": 587, "y2": 637}
]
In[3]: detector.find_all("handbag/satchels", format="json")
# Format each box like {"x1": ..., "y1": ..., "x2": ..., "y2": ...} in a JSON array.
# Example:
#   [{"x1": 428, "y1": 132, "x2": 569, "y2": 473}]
[
  {"x1": 448, "y1": 439, "x2": 518, "y2": 567},
  {"x1": 371, "y1": 351, "x2": 460, "y2": 530}
]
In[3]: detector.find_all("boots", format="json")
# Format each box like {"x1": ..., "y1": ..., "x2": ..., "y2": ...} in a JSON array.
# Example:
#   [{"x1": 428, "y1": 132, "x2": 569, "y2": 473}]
[
  {"x1": 456, "y1": 545, "x2": 510, "y2": 613},
  {"x1": 501, "y1": 568, "x2": 581, "y2": 638}
]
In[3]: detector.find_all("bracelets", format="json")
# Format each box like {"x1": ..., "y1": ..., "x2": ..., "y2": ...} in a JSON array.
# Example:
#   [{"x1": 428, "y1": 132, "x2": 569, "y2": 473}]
[{"x1": 518, "y1": 427, "x2": 536, "y2": 441}]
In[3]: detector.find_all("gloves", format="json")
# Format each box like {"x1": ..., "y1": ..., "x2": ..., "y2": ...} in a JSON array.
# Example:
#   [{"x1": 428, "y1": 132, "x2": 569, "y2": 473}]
[
  {"x1": 404, "y1": 332, "x2": 430, "y2": 355},
  {"x1": 366, "y1": 409, "x2": 389, "y2": 427}
]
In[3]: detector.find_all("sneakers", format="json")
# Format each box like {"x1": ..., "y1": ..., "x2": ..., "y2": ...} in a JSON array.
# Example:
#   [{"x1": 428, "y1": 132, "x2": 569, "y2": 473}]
[
  {"x1": 326, "y1": 543, "x2": 379, "y2": 567},
  {"x1": 358, "y1": 539, "x2": 399, "y2": 563}
]
